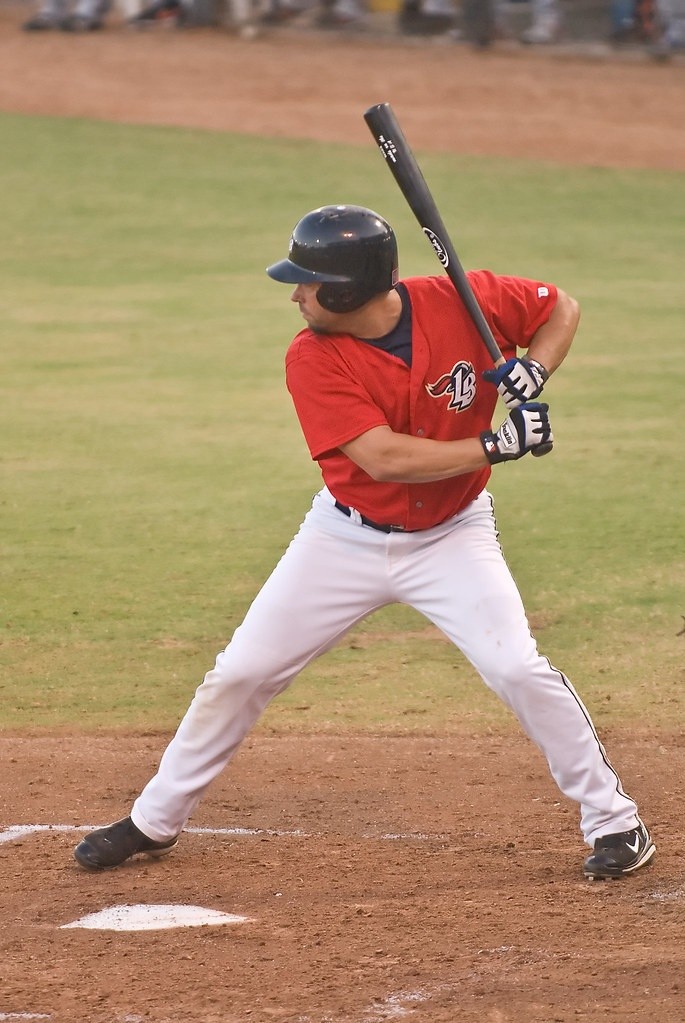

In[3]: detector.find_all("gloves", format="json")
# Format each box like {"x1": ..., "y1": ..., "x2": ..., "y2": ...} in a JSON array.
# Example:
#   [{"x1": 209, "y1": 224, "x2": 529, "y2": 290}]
[
  {"x1": 479, "y1": 402, "x2": 554, "y2": 464},
  {"x1": 482, "y1": 354, "x2": 549, "y2": 410}
]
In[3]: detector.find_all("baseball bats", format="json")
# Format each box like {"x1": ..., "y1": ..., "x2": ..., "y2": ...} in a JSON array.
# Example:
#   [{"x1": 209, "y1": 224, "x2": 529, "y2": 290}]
[{"x1": 362, "y1": 98, "x2": 555, "y2": 458}]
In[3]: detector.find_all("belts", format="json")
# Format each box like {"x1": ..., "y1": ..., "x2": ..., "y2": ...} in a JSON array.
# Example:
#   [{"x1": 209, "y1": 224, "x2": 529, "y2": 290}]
[{"x1": 334, "y1": 501, "x2": 405, "y2": 533}]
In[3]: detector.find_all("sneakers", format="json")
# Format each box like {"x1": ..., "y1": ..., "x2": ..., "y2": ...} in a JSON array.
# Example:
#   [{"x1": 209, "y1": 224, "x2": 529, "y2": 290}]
[
  {"x1": 583, "y1": 824, "x2": 656, "y2": 882},
  {"x1": 73, "y1": 816, "x2": 179, "y2": 873}
]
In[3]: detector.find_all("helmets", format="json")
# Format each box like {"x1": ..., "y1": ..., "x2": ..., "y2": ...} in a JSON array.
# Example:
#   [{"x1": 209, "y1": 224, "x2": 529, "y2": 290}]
[{"x1": 265, "y1": 204, "x2": 399, "y2": 313}]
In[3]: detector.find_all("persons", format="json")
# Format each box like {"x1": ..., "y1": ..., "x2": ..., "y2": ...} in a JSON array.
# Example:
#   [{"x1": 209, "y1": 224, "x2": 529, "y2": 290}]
[
  {"x1": 74, "y1": 205, "x2": 657, "y2": 881},
  {"x1": 24, "y1": 0, "x2": 685, "y2": 62}
]
